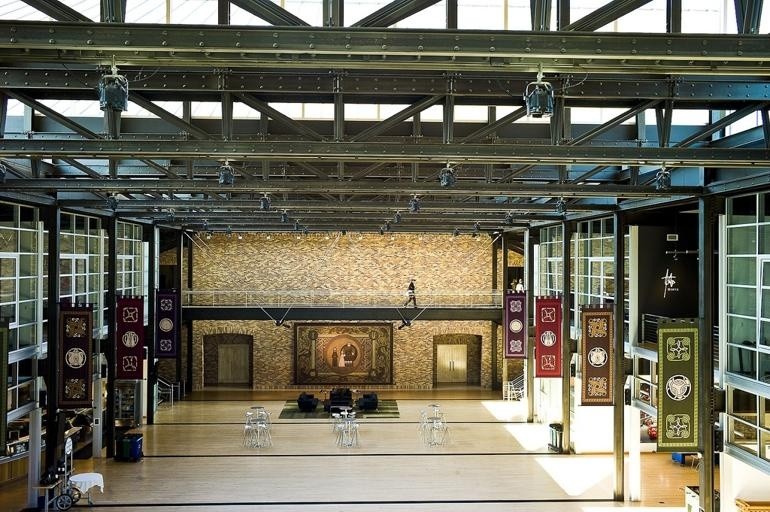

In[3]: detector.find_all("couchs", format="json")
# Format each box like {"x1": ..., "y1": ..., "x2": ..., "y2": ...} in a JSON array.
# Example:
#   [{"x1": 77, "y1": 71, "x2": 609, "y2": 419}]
[{"x1": 297, "y1": 387, "x2": 378, "y2": 412}]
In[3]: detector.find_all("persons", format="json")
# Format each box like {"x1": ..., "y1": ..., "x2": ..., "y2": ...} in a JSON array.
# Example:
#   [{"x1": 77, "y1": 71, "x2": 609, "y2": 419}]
[
  {"x1": 511, "y1": 279, "x2": 524, "y2": 293},
  {"x1": 402, "y1": 279, "x2": 418, "y2": 308}
]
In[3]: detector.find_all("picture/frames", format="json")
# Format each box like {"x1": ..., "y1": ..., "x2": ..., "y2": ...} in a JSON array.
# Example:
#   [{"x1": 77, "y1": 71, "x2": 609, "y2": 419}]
[{"x1": 293, "y1": 322, "x2": 394, "y2": 386}]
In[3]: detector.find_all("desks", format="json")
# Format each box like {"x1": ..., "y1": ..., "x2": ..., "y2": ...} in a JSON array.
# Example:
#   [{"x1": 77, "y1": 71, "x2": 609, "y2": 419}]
[
  {"x1": 68, "y1": 473, "x2": 105, "y2": 506},
  {"x1": 32, "y1": 480, "x2": 63, "y2": 512}
]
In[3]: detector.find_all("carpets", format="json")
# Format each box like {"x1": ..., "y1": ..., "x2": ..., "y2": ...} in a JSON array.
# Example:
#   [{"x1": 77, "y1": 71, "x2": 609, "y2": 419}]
[{"x1": 279, "y1": 400, "x2": 400, "y2": 419}]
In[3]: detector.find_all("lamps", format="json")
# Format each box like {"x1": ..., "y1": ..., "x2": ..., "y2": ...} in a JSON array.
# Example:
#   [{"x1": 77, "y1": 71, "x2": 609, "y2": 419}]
[
  {"x1": 554, "y1": 198, "x2": 569, "y2": 215},
  {"x1": 100, "y1": 65, "x2": 130, "y2": 116},
  {"x1": 159, "y1": 192, "x2": 519, "y2": 240},
  {"x1": 653, "y1": 167, "x2": 671, "y2": 195},
  {"x1": 105, "y1": 192, "x2": 120, "y2": 210},
  {"x1": 437, "y1": 167, "x2": 456, "y2": 190},
  {"x1": 522, "y1": 81, "x2": 557, "y2": 120},
  {"x1": 217, "y1": 161, "x2": 235, "y2": 186}
]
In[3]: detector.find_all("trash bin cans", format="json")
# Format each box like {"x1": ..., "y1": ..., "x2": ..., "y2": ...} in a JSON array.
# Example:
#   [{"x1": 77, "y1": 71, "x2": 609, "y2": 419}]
[
  {"x1": 548, "y1": 423, "x2": 563, "y2": 449},
  {"x1": 117, "y1": 433, "x2": 143, "y2": 463}
]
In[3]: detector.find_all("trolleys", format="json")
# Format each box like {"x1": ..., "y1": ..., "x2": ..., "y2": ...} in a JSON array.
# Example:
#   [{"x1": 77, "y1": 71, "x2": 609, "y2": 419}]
[{"x1": 57, "y1": 438, "x2": 81, "y2": 510}]
